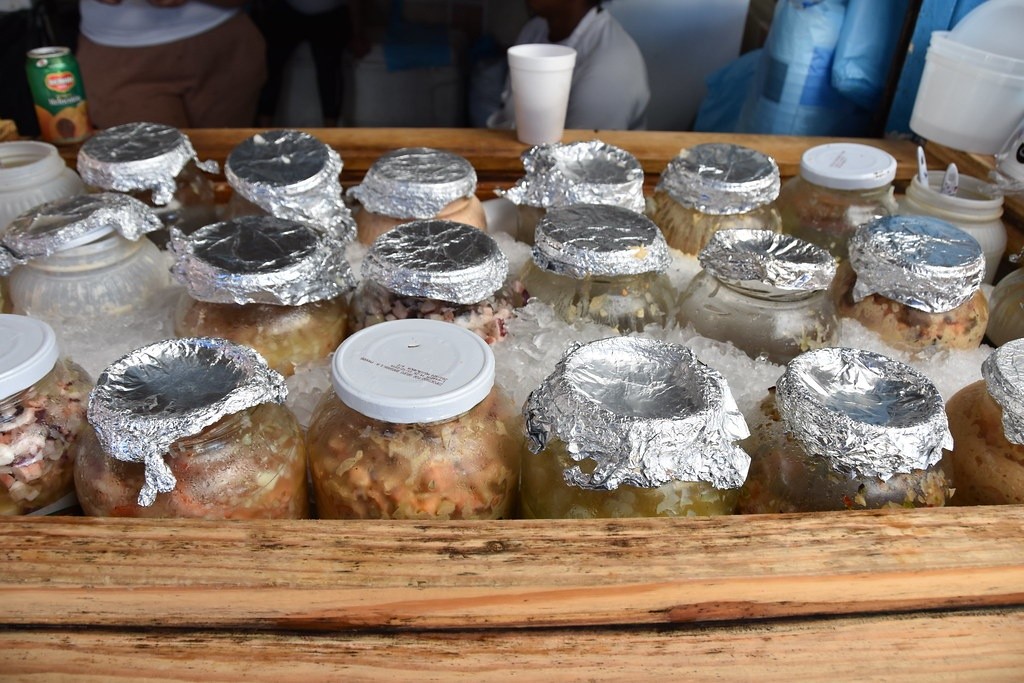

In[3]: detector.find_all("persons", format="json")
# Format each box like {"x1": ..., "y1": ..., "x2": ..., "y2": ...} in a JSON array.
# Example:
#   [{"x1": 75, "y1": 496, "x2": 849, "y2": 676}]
[
  {"x1": 405, "y1": 0, "x2": 651, "y2": 130},
  {"x1": 80, "y1": 0, "x2": 264, "y2": 131},
  {"x1": 246, "y1": 0, "x2": 381, "y2": 128}
]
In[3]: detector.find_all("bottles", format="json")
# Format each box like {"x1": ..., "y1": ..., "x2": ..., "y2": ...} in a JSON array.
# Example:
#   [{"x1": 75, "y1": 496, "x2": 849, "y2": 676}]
[{"x1": 0, "y1": 122, "x2": 1024, "y2": 520}]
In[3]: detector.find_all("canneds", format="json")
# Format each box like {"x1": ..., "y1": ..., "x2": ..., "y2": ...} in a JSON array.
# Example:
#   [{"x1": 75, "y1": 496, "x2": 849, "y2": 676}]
[{"x1": 26, "y1": 47, "x2": 90, "y2": 144}]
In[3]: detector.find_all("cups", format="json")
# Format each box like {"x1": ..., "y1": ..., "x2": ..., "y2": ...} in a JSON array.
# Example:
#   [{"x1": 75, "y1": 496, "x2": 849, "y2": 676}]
[{"x1": 508, "y1": 43, "x2": 578, "y2": 146}]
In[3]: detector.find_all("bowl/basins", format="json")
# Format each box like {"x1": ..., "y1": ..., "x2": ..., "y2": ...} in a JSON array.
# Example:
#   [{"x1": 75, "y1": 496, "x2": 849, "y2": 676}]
[{"x1": 910, "y1": 29, "x2": 1023, "y2": 154}]
